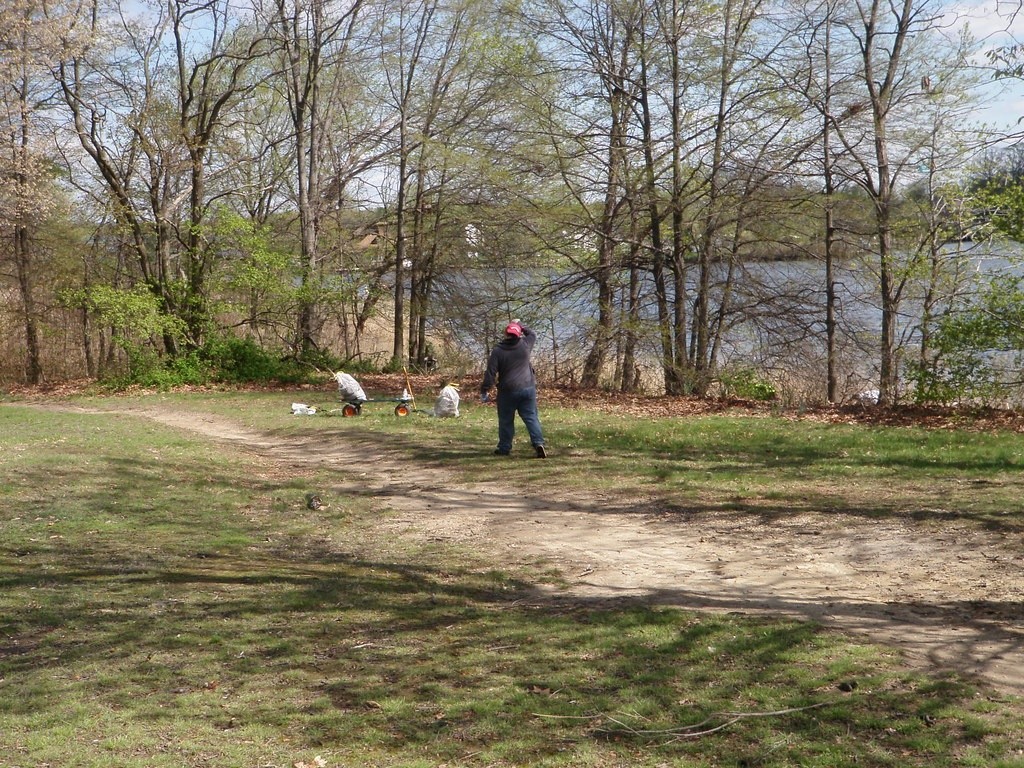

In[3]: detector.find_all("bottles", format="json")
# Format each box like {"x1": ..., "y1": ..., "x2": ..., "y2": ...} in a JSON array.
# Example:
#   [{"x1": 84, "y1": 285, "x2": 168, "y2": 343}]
[{"x1": 403, "y1": 389, "x2": 407, "y2": 398}]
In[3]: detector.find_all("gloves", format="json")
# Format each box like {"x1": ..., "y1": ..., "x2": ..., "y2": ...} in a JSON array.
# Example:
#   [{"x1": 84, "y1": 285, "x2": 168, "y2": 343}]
[
  {"x1": 482, "y1": 393, "x2": 490, "y2": 402},
  {"x1": 511, "y1": 318, "x2": 520, "y2": 324}
]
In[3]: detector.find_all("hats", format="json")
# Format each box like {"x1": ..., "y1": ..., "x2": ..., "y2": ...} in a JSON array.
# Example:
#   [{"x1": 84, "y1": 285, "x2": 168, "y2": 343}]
[{"x1": 506, "y1": 323, "x2": 522, "y2": 338}]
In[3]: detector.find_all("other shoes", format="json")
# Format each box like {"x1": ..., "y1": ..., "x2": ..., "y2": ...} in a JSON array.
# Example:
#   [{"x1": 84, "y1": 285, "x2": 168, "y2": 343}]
[
  {"x1": 494, "y1": 449, "x2": 509, "y2": 455},
  {"x1": 536, "y1": 445, "x2": 546, "y2": 458}
]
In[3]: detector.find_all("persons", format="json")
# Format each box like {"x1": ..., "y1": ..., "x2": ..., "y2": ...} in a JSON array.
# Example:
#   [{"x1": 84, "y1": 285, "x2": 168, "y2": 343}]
[{"x1": 480, "y1": 319, "x2": 548, "y2": 458}]
